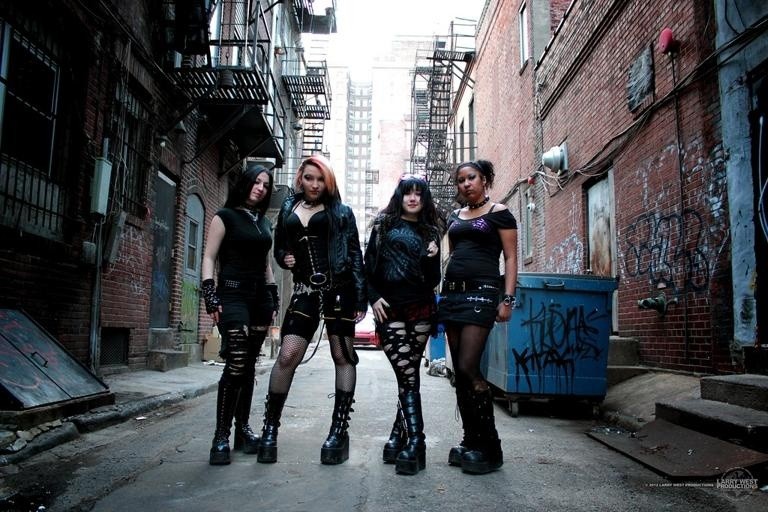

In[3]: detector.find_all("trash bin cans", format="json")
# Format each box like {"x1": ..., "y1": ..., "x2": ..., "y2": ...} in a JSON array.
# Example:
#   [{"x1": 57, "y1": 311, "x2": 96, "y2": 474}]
[{"x1": 424, "y1": 271, "x2": 619, "y2": 417}]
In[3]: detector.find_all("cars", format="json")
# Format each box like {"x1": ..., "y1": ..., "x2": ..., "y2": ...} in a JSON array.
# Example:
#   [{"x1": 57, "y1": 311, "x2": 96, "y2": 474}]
[{"x1": 352, "y1": 304, "x2": 383, "y2": 351}]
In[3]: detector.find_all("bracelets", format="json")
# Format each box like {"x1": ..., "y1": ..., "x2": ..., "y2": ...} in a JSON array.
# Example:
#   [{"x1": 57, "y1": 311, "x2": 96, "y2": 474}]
[
  {"x1": 503, "y1": 295, "x2": 517, "y2": 310},
  {"x1": 503, "y1": 295, "x2": 516, "y2": 306}
]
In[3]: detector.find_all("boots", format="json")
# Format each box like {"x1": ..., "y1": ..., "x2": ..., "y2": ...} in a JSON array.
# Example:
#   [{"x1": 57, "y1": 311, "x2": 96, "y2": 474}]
[
  {"x1": 448, "y1": 388, "x2": 503, "y2": 473},
  {"x1": 258, "y1": 395, "x2": 288, "y2": 463},
  {"x1": 210, "y1": 384, "x2": 240, "y2": 464},
  {"x1": 234, "y1": 384, "x2": 259, "y2": 453},
  {"x1": 383, "y1": 393, "x2": 425, "y2": 474},
  {"x1": 321, "y1": 391, "x2": 355, "y2": 465}
]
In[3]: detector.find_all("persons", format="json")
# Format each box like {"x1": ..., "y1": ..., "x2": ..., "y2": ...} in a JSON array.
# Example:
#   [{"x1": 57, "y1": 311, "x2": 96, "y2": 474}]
[
  {"x1": 440, "y1": 160, "x2": 517, "y2": 474},
  {"x1": 256, "y1": 156, "x2": 368, "y2": 465},
  {"x1": 364, "y1": 174, "x2": 441, "y2": 476},
  {"x1": 203, "y1": 165, "x2": 280, "y2": 464}
]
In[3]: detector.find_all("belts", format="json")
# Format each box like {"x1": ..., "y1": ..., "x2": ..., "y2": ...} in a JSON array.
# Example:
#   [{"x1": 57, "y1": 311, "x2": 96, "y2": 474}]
[{"x1": 442, "y1": 281, "x2": 497, "y2": 294}]
